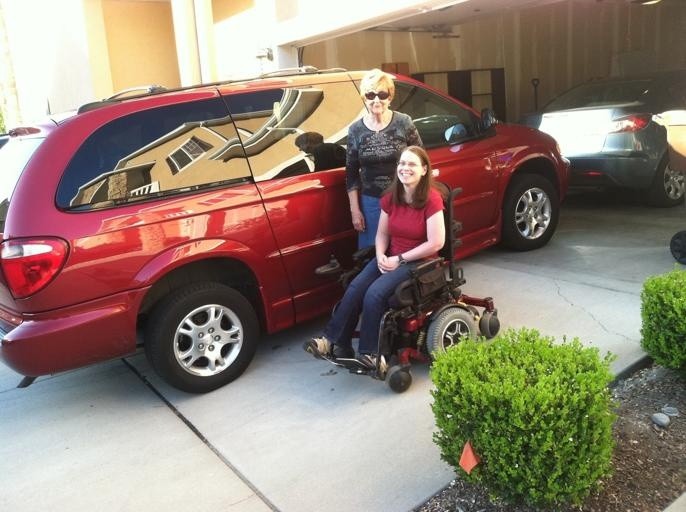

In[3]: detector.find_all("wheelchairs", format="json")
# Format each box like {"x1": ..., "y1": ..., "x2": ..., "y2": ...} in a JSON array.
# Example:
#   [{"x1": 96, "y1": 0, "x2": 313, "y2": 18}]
[{"x1": 306, "y1": 179, "x2": 502, "y2": 393}]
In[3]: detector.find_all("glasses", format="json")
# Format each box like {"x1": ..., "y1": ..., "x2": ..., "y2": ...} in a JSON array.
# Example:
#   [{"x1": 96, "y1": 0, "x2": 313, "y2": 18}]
[{"x1": 365, "y1": 90, "x2": 390, "y2": 100}]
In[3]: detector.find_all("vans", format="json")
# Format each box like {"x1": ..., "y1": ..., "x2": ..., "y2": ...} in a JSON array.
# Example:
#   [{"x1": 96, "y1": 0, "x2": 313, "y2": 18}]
[{"x1": 0, "y1": 66, "x2": 571, "y2": 395}]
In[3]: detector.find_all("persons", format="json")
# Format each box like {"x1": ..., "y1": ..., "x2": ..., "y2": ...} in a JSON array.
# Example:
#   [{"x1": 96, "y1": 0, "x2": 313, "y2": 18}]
[
  {"x1": 303, "y1": 145, "x2": 446, "y2": 381},
  {"x1": 344, "y1": 69, "x2": 424, "y2": 268},
  {"x1": 295, "y1": 132, "x2": 347, "y2": 172}
]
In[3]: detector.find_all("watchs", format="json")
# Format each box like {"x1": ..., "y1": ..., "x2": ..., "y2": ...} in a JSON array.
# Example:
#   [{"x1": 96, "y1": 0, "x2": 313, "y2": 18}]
[{"x1": 398, "y1": 254, "x2": 408, "y2": 266}]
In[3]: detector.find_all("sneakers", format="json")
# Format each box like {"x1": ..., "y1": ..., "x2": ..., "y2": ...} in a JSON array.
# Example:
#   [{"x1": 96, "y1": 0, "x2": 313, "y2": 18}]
[
  {"x1": 303, "y1": 337, "x2": 343, "y2": 355},
  {"x1": 355, "y1": 354, "x2": 387, "y2": 380}
]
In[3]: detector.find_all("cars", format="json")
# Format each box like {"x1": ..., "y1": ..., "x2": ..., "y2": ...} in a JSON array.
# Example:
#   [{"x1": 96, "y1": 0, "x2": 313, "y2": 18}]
[{"x1": 518, "y1": 72, "x2": 686, "y2": 206}]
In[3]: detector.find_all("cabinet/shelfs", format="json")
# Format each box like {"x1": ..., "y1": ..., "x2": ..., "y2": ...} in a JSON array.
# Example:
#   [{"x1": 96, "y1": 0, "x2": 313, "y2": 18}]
[{"x1": 413, "y1": 68, "x2": 505, "y2": 124}]
